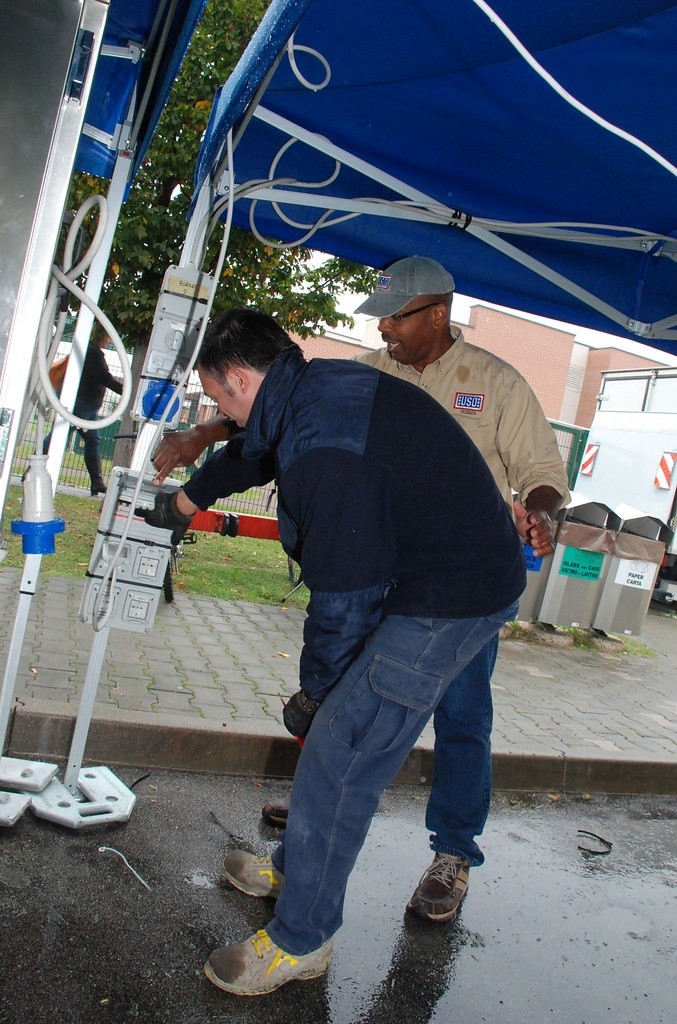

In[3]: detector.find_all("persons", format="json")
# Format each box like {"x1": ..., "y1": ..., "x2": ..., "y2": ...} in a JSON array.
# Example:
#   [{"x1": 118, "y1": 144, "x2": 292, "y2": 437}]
[
  {"x1": 20, "y1": 319, "x2": 108, "y2": 497},
  {"x1": 149, "y1": 256, "x2": 572, "y2": 922},
  {"x1": 132, "y1": 307, "x2": 528, "y2": 996}
]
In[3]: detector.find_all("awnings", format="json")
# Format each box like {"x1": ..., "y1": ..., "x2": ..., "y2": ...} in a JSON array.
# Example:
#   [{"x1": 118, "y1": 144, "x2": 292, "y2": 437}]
[{"x1": 1, "y1": 0, "x2": 677, "y2": 795}]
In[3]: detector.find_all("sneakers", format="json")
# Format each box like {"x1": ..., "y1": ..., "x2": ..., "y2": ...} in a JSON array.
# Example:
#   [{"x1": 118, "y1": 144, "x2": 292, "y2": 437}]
[
  {"x1": 261, "y1": 793, "x2": 291, "y2": 826},
  {"x1": 223, "y1": 849, "x2": 286, "y2": 899},
  {"x1": 203, "y1": 930, "x2": 334, "y2": 996},
  {"x1": 407, "y1": 852, "x2": 471, "y2": 922}
]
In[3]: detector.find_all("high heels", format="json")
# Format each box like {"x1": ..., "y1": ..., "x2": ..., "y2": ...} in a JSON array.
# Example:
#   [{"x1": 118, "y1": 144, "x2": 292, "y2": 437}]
[{"x1": 91, "y1": 486, "x2": 107, "y2": 495}]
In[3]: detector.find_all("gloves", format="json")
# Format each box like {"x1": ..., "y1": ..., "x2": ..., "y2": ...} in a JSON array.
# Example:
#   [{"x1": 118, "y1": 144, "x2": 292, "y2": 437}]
[
  {"x1": 135, "y1": 492, "x2": 197, "y2": 547},
  {"x1": 283, "y1": 689, "x2": 321, "y2": 737}
]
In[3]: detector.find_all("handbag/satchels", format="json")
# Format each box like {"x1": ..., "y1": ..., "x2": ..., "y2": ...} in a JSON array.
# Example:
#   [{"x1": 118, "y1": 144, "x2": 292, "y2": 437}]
[{"x1": 49, "y1": 356, "x2": 69, "y2": 389}]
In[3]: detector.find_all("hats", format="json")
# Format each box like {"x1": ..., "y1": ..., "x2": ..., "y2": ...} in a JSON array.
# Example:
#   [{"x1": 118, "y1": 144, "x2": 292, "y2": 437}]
[{"x1": 354, "y1": 255, "x2": 455, "y2": 318}]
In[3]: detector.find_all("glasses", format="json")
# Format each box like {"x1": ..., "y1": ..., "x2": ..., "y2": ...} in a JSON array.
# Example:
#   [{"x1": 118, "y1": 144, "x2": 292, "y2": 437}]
[{"x1": 386, "y1": 302, "x2": 438, "y2": 325}]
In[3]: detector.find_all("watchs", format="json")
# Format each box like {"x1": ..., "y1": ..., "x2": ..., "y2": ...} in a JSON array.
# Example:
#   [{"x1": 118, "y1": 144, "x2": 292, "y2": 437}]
[{"x1": 299, "y1": 691, "x2": 319, "y2": 713}]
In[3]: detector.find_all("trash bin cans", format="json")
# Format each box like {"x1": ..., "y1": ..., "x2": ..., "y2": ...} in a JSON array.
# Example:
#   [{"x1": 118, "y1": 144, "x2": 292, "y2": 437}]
[
  {"x1": 593, "y1": 515, "x2": 675, "y2": 637},
  {"x1": 511, "y1": 493, "x2": 557, "y2": 620},
  {"x1": 533, "y1": 501, "x2": 621, "y2": 631}
]
